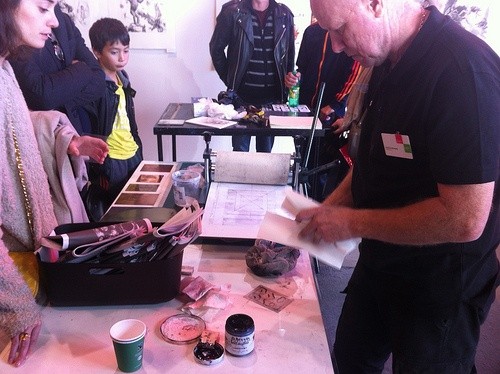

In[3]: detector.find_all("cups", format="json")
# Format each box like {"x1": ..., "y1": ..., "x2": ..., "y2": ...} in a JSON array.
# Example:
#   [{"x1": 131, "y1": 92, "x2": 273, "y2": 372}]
[
  {"x1": 170, "y1": 169, "x2": 201, "y2": 207},
  {"x1": 109, "y1": 319, "x2": 146, "y2": 373}
]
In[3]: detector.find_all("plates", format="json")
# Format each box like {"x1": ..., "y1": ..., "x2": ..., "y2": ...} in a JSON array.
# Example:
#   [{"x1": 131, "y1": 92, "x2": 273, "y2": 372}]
[{"x1": 160, "y1": 314, "x2": 206, "y2": 345}]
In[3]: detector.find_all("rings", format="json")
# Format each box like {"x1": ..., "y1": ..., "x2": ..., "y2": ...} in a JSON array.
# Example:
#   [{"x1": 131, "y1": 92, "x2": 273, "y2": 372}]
[{"x1": 19, "y1": 333, "x2": 29, "y2": 340}]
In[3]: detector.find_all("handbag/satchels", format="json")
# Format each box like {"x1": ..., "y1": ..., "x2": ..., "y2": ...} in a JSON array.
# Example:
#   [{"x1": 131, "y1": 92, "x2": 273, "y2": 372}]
[{"x1": 7, "y1": 251, "x2": 40, "y2": 300}]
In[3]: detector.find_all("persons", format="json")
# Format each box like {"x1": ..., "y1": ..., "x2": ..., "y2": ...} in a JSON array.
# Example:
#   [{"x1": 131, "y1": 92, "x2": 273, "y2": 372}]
[
  {"x1": 89, "y1": 18, "x2": 144, "y2": 212},
  {"x1": 296, "y1": 0, "x2": 500, "y2": 374},
  {"x1": 285, "y1": 24, "x2": 375, "y2": 191},
  {"x1": 209, "y1": 0, "x2": 294, "y2": 153},
  {"x1": 0, "y1": 0, "x2": 110, "y2": 367},
  {"x1": 8, "y1": 3, "x2": 106, "y2": 134}
]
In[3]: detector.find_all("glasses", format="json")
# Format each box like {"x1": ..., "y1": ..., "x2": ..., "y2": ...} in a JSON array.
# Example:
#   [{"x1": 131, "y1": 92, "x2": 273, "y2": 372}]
[{"x1": 47, "y1": 32, "x2": 65, "y2": 61}]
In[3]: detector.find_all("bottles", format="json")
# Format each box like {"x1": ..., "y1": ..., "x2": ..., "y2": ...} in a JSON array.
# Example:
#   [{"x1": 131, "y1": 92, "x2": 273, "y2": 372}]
[{"x1": 288, "y1": 70, "x2": 300, "y2": 107}]
